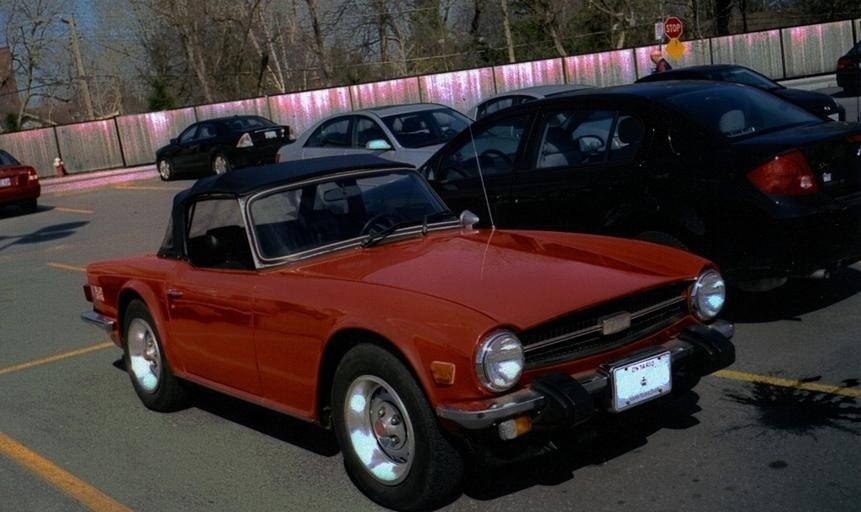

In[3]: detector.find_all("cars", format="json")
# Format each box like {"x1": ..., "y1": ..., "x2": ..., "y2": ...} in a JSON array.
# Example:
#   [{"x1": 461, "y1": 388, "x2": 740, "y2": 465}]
[
  {"x1": 834, "y1": 44, "x2": 861, "y2": 94},
  {"x1": 633, "y1": 63, "x2": 845, "y2": 124},
  {"x1": 155, "y1": 116, "x2": 295, "y2": 180},
  {"x1": 79, "y1": 154, "x2": 736, "y2": 510},
  {"x1": 0, "y1": 149, "x2": 40, "y2": 213},
  {"x1": 469, "y1": 82, "x2": 629, "y2": 152},
  {"x1": 352, "y1": 81, "x2": 861, "y2": 310},
  {"x1": 267, "y1": 103, "x2": 568, "y2": 199}
]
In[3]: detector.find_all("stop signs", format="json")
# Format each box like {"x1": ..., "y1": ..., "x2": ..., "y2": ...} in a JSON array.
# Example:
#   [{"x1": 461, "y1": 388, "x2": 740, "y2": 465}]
[{"x1": 663, "y1": 17, "x2": 684, "y2": 40}]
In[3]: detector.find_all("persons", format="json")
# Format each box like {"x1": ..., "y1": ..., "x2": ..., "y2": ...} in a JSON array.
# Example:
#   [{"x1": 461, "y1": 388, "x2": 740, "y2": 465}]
[{"x1": 650, "y1": 47, "x2": 672, "y2": 71}]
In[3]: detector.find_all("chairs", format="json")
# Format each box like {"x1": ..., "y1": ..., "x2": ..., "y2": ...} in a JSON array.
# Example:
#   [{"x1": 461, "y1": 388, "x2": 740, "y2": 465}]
[
  {"x1": 206, "y1": 224, "x2": 260, "y2": 264},
  {"x1": 301, "y1": 208, "x2": 354, "y2": 248}
]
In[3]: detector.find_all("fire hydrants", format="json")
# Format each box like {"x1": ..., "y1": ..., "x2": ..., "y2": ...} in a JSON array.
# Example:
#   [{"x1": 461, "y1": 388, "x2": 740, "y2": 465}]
[{"x1": 54, "y1": 157, "x2": 65, "y2": 176}]
